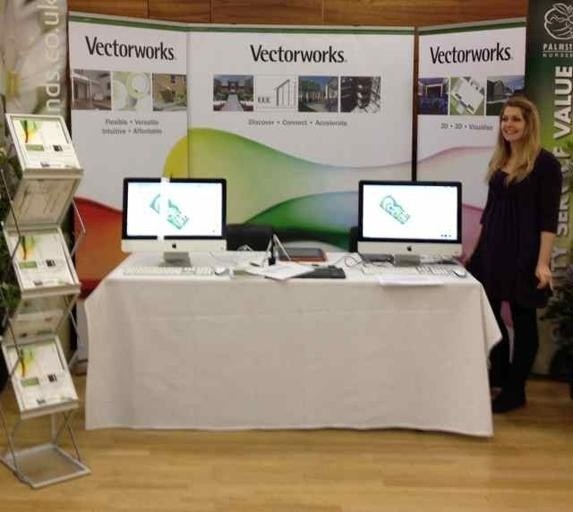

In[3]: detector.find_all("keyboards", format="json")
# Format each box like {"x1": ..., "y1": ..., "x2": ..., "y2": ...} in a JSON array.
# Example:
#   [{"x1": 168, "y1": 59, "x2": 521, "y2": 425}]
[
  {"x1": 125, "y1": 266, "x2": 213, "y2": 277},
  {"x1": 292, "y1": 268, "x2": 345, "y2": 278},
  {"x1": 362, "y1": 265, "x2": 450, "y2": 277}
]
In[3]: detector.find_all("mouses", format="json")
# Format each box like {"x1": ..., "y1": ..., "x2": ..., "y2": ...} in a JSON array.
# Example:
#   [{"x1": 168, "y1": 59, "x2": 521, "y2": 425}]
[
  {"x1": 453, "y1": 266, "x2": 467, "y2": 277},
  {"x1": 215, "y1": 266, "x2": 226, "y2": 274}
]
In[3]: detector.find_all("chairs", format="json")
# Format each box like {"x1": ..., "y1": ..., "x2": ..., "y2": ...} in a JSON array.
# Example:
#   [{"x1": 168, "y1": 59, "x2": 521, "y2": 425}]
[
  {"x1": 349, "y1": 226, "x2": 360, "y2": 254},
  {"x1": 216, "y1": 224, "x2": 288, "y2": 251}
]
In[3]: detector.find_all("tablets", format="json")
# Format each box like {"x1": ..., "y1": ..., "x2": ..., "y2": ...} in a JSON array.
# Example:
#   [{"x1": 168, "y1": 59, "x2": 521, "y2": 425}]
[{"x1": 278, "y1": 247, "x2": 325, "y2": 260}]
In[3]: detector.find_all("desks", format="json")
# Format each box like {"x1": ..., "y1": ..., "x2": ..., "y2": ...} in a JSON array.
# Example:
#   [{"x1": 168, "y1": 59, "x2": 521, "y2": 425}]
[{"x1": 85, "y1": 250, "x2": 504, "y2": 439}]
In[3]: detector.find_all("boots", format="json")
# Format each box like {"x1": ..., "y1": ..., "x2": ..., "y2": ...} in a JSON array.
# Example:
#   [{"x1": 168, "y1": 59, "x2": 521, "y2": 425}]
[{"x1": 487, "y1": 355, "x2": 529, "y2": 412}]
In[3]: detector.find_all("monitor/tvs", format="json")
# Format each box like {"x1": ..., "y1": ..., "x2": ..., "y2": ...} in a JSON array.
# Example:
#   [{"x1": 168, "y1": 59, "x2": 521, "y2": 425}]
[
  {"x1": 357, "y1": 180, "x2": 462, "y2": 265},
  {"x1": 121, "y1": 178, "x2": 227, "y2": 266}
]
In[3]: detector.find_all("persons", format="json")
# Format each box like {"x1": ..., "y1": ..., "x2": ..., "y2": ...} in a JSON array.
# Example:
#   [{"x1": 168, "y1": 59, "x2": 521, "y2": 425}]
[{"x1": 462, "y1": 94, "x2": 561, "y2": 414}]
